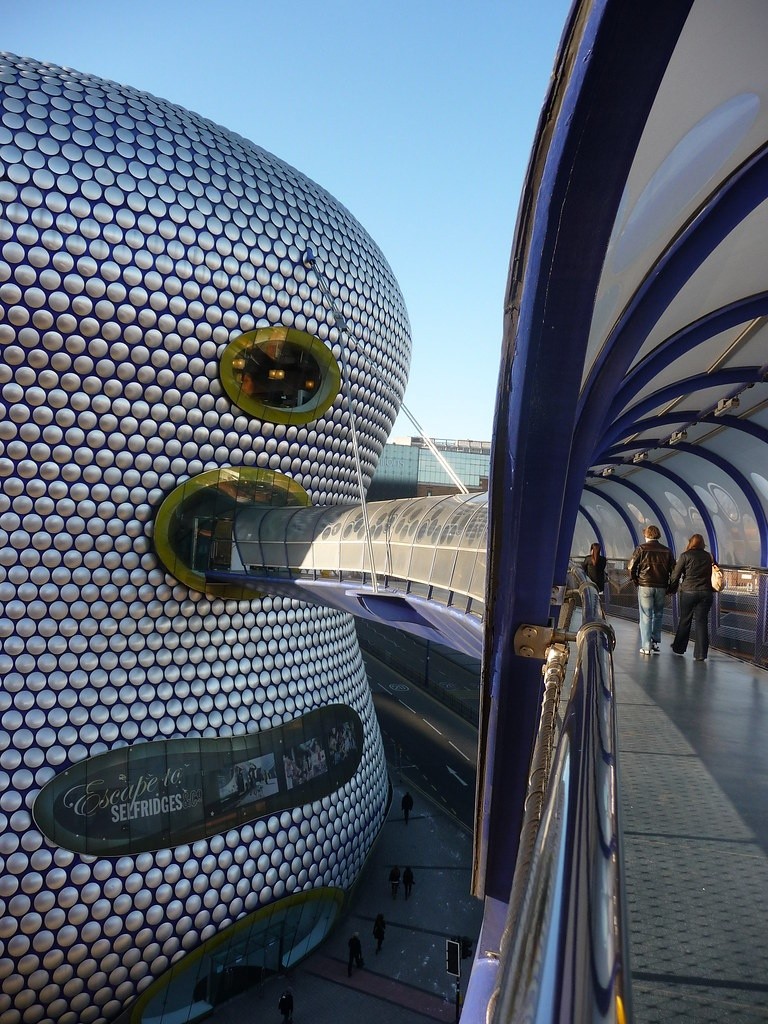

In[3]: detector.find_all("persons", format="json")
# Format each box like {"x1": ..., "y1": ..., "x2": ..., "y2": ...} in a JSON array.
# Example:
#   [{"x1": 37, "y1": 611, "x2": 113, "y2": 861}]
[
  {"x1": 403, "y1": 865, "x2": 415, "y2": 900},
  {"x1": 402, "y1": 792, "x2": 413, "y2": 824},
  {"x1": 582, "y1": 543, "x2": 606, "y2": 604},
  {"x1": 668, "y1": 533, "x2": 718, "y2": 661},
  {"x1": 348, "y1": 932, "x2": 362, "y2": 977},
  {"x1": 373, "y1": 913, "x2": 386, "y2": 954},
  {"x1": 627, "y1": 525, "x2": 674, "y2": 655},
  {"x1": 389, "y1": 865, "x2": 400, "y2": 899},
  {"x1": 279, "y1": 987, "x2": 293, "y2": 1024}
]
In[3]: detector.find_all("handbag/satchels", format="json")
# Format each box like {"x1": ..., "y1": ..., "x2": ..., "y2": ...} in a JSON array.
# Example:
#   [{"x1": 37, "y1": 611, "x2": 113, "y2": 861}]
[{"x1": 709, "y1": 552, "x2": 725, "y2": 592}]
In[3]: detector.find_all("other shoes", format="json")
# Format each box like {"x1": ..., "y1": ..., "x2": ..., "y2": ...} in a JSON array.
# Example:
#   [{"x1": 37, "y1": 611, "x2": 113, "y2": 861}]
[
  {"x1": 639, "y1": 648, "x2": 651, "y2": 654},
  {"x1": 651, "y1": 641, "x2": 661, "y2": 654},
  {"x1": 670, "y1": 644, "x2": 684, "y2": 656},
  {"x1": 693, "y1": 656, "x2": 704, "y2": 661}
]
